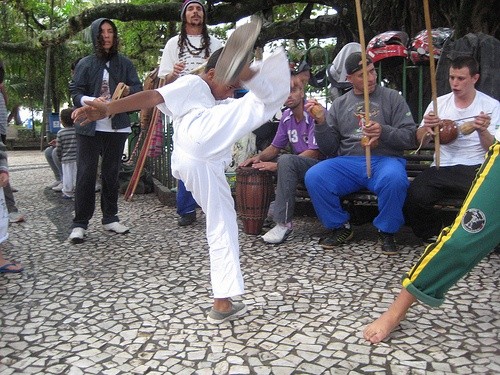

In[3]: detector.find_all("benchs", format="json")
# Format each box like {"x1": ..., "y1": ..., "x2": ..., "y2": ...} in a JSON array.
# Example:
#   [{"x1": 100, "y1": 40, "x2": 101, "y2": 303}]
[{"x1": 295, "y1": 137, "x2": 467, "y2": 213}]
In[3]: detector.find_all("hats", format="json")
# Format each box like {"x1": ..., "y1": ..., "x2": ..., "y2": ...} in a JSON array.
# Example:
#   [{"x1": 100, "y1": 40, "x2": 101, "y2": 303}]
[
  {"x1": 290, "y1": 59, "x2": 310, "y2": 76},
  {"x1": 345, "y1": 53, "x2": 372, "y2": 75},
  {"x1": 180, "y1": 0, "x2": 206, "y2": 22}
]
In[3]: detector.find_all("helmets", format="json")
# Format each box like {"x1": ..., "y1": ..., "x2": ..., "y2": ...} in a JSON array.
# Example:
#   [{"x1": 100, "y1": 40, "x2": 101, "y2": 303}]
[
  {"x1": 409, "y1": 29, "x2": 446, "y2": 62},
  {"x1": 365, "y1": 30, "x2": 408, "y2": 64}
]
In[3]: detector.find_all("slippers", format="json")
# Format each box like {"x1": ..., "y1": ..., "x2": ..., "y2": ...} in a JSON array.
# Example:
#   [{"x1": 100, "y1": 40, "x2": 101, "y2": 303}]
[{"x1": 0, "y1": 260, "x2": 24, "y2": 274}]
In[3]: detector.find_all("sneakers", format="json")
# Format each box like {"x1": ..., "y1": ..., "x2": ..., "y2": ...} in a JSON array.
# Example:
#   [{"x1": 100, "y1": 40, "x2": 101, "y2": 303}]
[
  {"x1": 8, "y1": 211, "x2": 24, "y2": 222},
  {"x1": 103, "y1": 222, "x2": 130, "y2": 234},
  {"x1": 377, "y1": 228, "x2": 398, "y2": 254},
  {"x1": 69, "y1": 227, "x2": 88, "y2": 242},
  {"x1": 260, "y1": 223, "x2": 295, "y2": 245},
  {"x1": 319, "y1": 224, "x2": 354, "y2": 248}
]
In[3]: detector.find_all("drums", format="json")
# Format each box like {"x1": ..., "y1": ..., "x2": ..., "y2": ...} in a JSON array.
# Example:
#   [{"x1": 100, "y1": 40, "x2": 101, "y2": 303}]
[{"x1": 235, "y1": 166, "x2": 272, "y2": 235}]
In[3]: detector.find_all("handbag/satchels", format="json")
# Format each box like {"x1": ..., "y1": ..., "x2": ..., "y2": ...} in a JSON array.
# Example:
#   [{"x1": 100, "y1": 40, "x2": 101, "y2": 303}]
[{"x1": 117, "y1": 165, "x2": 152, "y2": 194}]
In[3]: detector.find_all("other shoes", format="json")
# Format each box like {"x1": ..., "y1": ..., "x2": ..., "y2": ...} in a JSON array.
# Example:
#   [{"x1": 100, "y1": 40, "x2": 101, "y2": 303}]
[
  {"x1": 46, "y1": 179, "x2": 59, "y2": 189},
  {"x1": 53, "y1": 182, "x2": 63, "y2": 191},
  {"x1": 177, "y1": 210, "x2": 196, "y2": 227},
  {"x1": 206, "y1": 298, "x2": 248, "y2": 324},
  {"x1": 213, "y1": 18, "x2": 262, "y2": 85}
]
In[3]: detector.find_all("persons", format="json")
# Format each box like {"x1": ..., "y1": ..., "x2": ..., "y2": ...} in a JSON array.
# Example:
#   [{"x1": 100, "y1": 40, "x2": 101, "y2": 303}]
[
  {"x1": 69, "y1": 17, "x2": 143, "y2": 243},
  {"x1": 71, "y1": 13, "x2": 290, "y2": 325},
  {"x1": 400, "y1": 54, "x2": 500, "y2": 248},
  {"x1": 57, "y1": 108, "x2": 78, "y2": 199},
  {"x1": 239, "y1": 66, "x2": 328, "y2": 243},
  {"x1": 0, "y1": 59, "x2": 24, "y2": 271},
  {"x1": 45, "y1": 139, "x2": 63, "y2": 191},
  {"x1": 158, "y1": 0, "x2": 225, "y2": 227},
  {"x1": 304, "y1": 51, "x2": 417, "y2": 254},
  {"x1": 362, "y1": 122, "x2": 500, "y2": 342}
]
